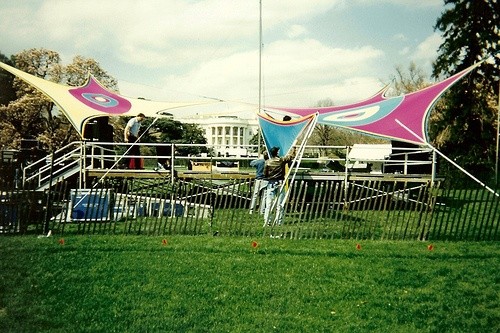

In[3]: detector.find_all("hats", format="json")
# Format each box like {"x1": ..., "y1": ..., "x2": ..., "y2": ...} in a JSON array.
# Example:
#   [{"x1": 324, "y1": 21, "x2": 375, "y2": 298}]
[{"x1": 270, "y1": 147, "x2": 280, "y2": 156}]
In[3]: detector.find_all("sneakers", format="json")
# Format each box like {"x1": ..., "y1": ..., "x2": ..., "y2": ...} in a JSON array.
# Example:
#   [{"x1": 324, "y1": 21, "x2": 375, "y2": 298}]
[
  {"x1": 276, "y1": 218, "x2": 282, "y2": 224},
  {"x1": 261, "y1": 208, "x2": 265, "y2": 214},
  {"x1": 264, "y1": 219, "x2": 271, "y2": 226},
  {"x1": 250, "y1": 210, "x2": 253, "y2": 214}
]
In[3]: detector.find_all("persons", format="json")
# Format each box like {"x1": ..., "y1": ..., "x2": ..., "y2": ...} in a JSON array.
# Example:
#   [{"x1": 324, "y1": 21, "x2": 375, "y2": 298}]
[
  {"x1": 262, "y1": 147, "x2": 293, "y2": 228},
  {"x1": 124, "y1": 112, "x2": 146, "y2": 170},
  {"x1": 249, "y1": 152, "x2": 269, "y2": 215}
]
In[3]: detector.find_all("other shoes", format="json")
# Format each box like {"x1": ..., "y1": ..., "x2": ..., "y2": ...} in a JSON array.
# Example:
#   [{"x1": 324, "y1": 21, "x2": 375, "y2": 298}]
[
  {"x1": 136, "y1": 167, "x2": 145, "y2": 170},
  {"x1": 122, "y1": 164, "x2": 128, "y2": 169}
]
